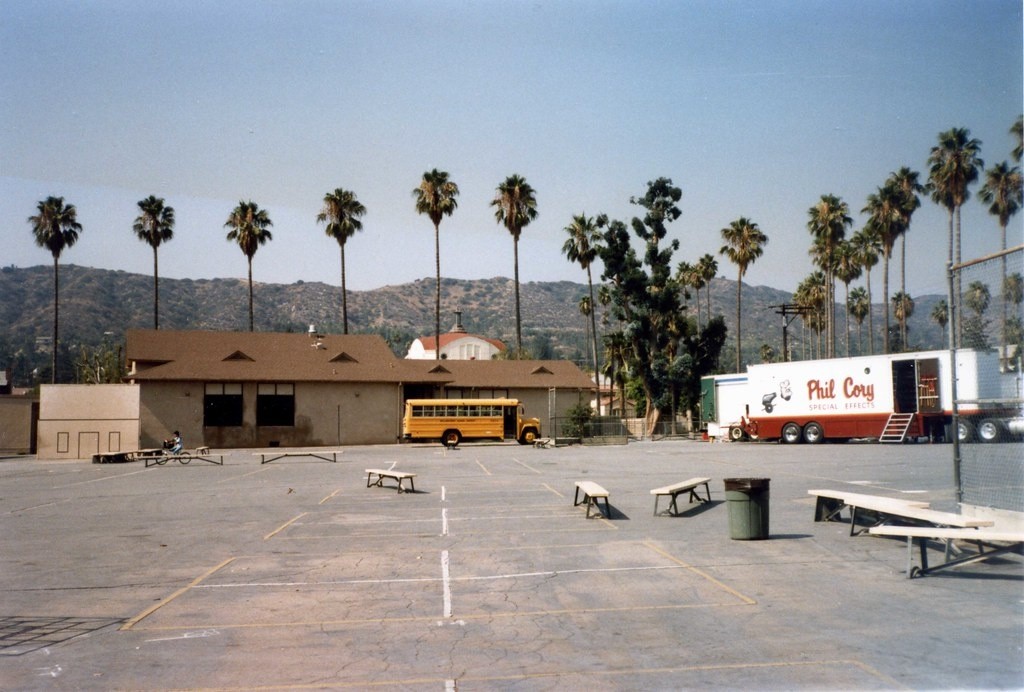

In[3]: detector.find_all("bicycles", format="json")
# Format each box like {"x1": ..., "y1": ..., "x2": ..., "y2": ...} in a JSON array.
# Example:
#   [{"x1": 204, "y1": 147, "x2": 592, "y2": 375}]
[{"x1": 156, "y1": 442, "x2": 191, "y2": 465}]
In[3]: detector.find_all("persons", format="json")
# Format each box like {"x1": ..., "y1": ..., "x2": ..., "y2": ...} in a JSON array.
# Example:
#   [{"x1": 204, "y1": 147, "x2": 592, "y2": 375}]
[{"x1": 169, "y1": 430, "x2": 183, "y2": 461}]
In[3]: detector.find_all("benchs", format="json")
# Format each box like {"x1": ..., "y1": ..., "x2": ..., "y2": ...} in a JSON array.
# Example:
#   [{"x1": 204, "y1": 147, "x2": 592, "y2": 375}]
[
  {"x1": 570, "y1": 477, "x2": 615, "y2": 523},
  {"x1": 252, "y1": 446, "x2": 344, "y2": 465},
  {"x1": 531, "y1": 437, "x2": 544, "y2": 448},
  {"x1": 364, "y1": 466, "x2": 417, "y2": 496},
  {"x1": 448, "y1": 439, "x2": 456, "y2": 450},
  {"x1": 90, "y1": 445, "x2": 225, "y2": 468},
  {"x1": 647, "y1": 475, "x2": 716, "y2": 521},
  {"x1": 803, "y1": 485, "x2": 1024, "y2": 584}
]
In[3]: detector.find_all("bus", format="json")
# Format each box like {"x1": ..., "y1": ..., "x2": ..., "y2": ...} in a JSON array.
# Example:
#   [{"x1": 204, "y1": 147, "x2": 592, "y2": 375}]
[{"x1": 402, "y1": 399, "x2": 540, "y2": 445}]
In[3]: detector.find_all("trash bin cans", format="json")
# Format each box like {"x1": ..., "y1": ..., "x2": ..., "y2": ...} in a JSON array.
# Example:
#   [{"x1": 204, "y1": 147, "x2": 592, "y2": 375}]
[{"x1": 725, "y1": 476, "x2": 772, "y2": 540}]
